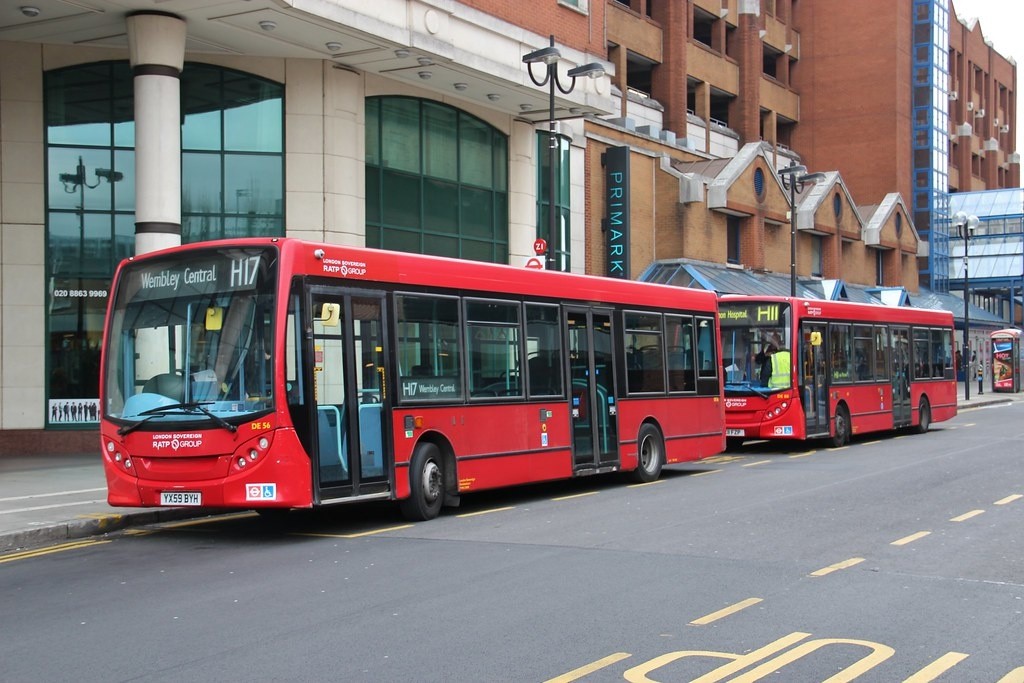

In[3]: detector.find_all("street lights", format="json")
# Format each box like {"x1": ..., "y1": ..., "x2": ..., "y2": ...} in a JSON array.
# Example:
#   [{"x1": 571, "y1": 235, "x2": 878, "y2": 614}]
[
  {"x1": 953, "y1": 211, "x2": 980, "y2": 400},
  {"x1": 778, "y1": 158, "x2": 826, "y2": 297},
  {"x1": 521, "y1": 35, "x2": 606, "y2": 270},
  {"x1": 58, "y1": 156, "x2": 123, "y2": 355}
]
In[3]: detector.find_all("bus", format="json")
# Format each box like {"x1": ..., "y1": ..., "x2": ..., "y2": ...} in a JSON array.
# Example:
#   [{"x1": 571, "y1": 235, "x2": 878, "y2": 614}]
[
  {"x1": 100, "y1": 238, "x2": 728, "y2": 522},
  {"x1": 705, "y1": 295, "x2": 958, "y2": 452}
]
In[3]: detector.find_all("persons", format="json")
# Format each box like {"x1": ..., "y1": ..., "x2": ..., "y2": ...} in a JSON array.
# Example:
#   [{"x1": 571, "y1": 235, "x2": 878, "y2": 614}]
[{"x1": 757, "y1": 335, "x2": 791, "y2": 391}]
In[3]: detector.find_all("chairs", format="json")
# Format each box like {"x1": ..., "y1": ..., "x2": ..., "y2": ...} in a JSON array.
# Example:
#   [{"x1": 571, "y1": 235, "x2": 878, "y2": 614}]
[{"x1": 473, "y1": 339, "x2": 686, "y2": 397}]
